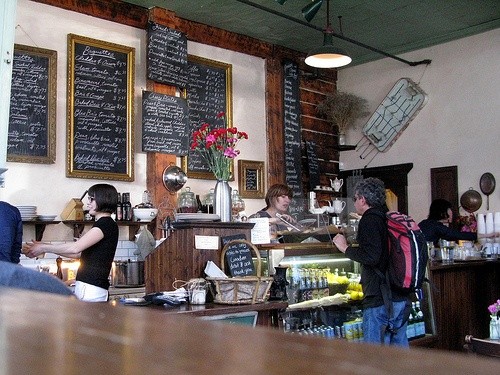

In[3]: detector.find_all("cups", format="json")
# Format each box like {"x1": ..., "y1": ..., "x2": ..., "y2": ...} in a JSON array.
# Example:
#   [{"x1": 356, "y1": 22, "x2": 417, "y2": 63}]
[
  {"x1": 313, "y1": 206, "x2": 334, "y2": 214},
  {"x1": 316, "y1": 186, "x2": 333, "y2": 190},
  {"x1": 426, "y1": 239, "x2": 500, "y2": 263}
]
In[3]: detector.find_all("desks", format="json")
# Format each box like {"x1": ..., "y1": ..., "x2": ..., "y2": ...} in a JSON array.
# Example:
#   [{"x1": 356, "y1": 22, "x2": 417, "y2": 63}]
[{"x1": 0, "y1": 287, "x2": 499, "y2": 375}]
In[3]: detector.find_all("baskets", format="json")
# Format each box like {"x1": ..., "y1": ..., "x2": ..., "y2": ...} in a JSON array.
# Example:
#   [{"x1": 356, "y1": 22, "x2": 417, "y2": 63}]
[{"x1": 205, "y1": 239, "x2": 274, "y2": 304}]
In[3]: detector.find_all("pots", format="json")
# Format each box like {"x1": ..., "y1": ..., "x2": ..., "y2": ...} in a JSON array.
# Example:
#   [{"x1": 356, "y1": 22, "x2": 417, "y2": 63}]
[
  {"x1": 459, "y1": 187, "x2": 482, "y2": 215},
  {"x1": 110, "y1": 260, "x2": 145, "y2": 288},
  {"x1": 162, "y1": 161, "x2": 187, "y2": 195},
  {"x1": 479, "y1": 172, "x2": 496, "y2": 210}
]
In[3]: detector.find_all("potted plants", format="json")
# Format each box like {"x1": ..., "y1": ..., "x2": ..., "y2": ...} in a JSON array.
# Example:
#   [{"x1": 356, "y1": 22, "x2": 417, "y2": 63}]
[{"x1": 313, "y1": 90, "x2": 371, "y2": 145}]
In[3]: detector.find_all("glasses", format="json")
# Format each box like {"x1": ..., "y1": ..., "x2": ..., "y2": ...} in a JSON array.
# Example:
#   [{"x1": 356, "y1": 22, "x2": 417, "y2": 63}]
[
  {"x1": 352, "y1": 197, "x2": 363, "y2": 202},
  {"x1": 88, "y1": 197, "x2": 94, "y2": 202}
]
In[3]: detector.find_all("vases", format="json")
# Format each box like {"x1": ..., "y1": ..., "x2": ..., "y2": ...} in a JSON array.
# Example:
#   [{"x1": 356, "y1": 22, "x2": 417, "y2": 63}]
[
  {"x1": 213, "y1": 180, "x2": 231, "y2": 222},
  {"x1": 489, "y1": 316, "x2": 499, "y2": 340}
]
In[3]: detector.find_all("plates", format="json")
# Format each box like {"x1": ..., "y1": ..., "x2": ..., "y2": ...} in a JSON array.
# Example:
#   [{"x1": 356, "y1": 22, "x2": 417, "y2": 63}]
[{"x1": 16, "y1": 205, "x2": 57, "y2": 220}]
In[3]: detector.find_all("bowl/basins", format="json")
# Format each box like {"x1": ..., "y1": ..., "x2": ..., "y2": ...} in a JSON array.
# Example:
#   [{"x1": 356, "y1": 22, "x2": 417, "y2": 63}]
[{"x1": 133, "y1": 209, "x2": 158, "y2": 222}]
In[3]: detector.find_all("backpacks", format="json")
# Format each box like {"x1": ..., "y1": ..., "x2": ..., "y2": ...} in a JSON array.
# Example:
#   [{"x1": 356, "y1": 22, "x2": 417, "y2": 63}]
[{"x1": 363, "y1": 208, "x2": 427, "y2": 290}]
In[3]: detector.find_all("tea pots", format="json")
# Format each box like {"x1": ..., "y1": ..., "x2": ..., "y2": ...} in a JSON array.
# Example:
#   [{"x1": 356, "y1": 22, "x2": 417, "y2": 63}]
[
  {"x1": 330, "y1": 178, "x2": 343, "y2": 192},
  {"x1": 328, "y1": 198, "x2": 346, "y2": 213}
]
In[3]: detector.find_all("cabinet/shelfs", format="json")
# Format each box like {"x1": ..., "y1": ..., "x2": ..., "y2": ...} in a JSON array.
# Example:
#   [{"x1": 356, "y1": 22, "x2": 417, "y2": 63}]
[{"x1": 21, "y1": 221, "x2": 499, "y2": 352}]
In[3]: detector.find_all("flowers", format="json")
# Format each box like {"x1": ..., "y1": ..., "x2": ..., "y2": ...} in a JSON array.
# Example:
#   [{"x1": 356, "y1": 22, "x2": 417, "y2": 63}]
[
  {"x1": 190, "y1": 112, "x2": 249, "y2": 180},
  {"x1": 487, "y1": 299, "x2": 500, "y2": 316}
]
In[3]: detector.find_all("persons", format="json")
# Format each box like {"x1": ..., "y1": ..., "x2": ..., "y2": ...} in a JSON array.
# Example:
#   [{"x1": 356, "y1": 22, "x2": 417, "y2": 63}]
[
  {"x1": 248, "y1": 183, "x2": 299, "y2": 243},
  {"x1": 418, "y1": 198, "x2": 499, "y2": 246},
  {"x1": 0, "y1": 201, "x2": 23, "y2": 264},
  {"x1": 23, "y1": 184, "x2": 119, "y2": 303},
  {"x1": 334, "y1": 178, "x2": 429, "y2": 349}
]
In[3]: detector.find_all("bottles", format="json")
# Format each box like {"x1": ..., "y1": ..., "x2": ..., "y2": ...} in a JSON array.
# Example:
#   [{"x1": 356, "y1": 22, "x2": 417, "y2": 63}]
[
  {"x1": 288, "y1": 267, "x2": 363, "y2": 301},
  {"x1": 188, "y1": 278, "x2": 207, "y2": 304},
  {"x1": 206, "y1": 188, "x2": 215, "y2": 213},
  {"x1": 179, "y1": 192, "x2": 197, "y2": 213},
  {"x1": 290, "y1": 315, "x2": 364, "y2": 344},
  {"x1": 116, "y1": 192, "x2": 132, "y2": 221},
  {"x1": 231, "y1": 189, "x2": 245, "y2": 214}
]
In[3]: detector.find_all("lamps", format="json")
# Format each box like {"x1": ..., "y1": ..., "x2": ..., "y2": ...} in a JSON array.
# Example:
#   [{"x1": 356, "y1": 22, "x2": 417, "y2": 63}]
[{"x1": 304, "y1": 0, "x2": 352, "y2": 69}]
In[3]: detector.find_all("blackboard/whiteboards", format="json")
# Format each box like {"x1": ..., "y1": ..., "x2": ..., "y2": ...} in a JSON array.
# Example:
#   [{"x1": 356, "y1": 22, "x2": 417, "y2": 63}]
[
  {"x1": 182, "y1": 53, "x2": 234, "y2": 182},
  {"x1": 280, "y1": 54, "x2": 322, "y2": 197},
  {"x1": 146, "y1": 20, "x2": 190, "y2": 89},
  {"x1": 65, "y1": 31, "x2": 136, "y2": 183},
  {"x1": 200, "y1": 310, "x2": 259, "y2": 327},
  {"x1": 7, "y1": 43, "x2": 59, "y2": 165},
  {"x1": 221, "y1": 233, "x2": 255, "y2": 277},
  {"x1": 139, "y1": 89, "x2": 191, "y2": 155}
]
